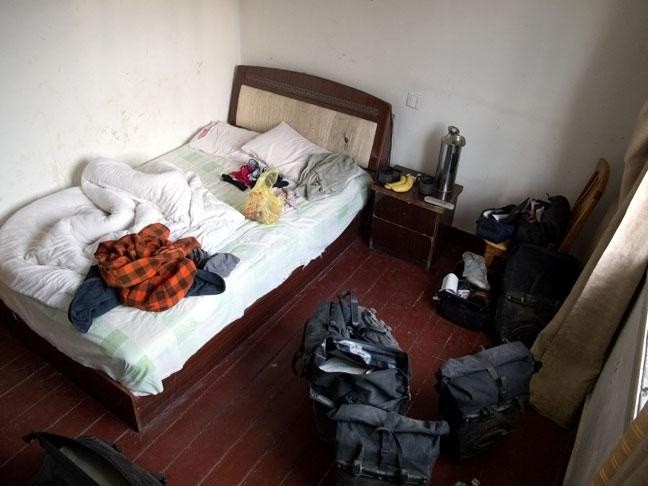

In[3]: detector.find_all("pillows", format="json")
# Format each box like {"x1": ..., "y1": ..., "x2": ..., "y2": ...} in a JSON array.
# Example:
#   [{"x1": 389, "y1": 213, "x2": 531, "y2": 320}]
[
  {"x1": 246, "y1": 122, "x2": 367, "y2": 194},
  {"x1": 189, "y1": 119, "x2": 269, "y2": 168}
]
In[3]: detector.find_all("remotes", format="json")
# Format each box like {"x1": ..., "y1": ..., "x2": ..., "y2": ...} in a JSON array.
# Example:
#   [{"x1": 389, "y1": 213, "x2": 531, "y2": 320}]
[{"x1": 424, "y1": 194, "x2": 454, "y2": 210}]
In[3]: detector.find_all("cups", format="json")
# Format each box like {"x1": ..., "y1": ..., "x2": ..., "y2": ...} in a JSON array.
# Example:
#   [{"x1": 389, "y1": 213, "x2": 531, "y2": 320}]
[
  {"x1": 376, "y1": 165, "x2": 394, "y2": 184},
  {"x1": 414, "y1": 172, "x2": 434, "y2": 195}
]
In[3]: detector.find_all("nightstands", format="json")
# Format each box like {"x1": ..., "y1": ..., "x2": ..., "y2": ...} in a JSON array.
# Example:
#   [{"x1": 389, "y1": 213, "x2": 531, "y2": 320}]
[{"x1": 371, "y1": 164, "x2": 464, "y2": 271}]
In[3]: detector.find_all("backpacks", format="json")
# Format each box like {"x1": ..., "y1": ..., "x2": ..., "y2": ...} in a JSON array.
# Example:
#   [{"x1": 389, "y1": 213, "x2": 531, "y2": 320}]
[
  {"x1": 290, "y1": 288, "x2": 413, "y2": 448},
  {"x1": 433, "y1": 335, "x2": 538, "y2": 462}
]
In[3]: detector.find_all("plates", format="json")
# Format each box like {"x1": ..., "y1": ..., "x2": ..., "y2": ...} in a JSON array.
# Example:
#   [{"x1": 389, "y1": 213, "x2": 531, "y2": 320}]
[{"x1": 380, "y1": 170, "x2": 400, "y2": 185}]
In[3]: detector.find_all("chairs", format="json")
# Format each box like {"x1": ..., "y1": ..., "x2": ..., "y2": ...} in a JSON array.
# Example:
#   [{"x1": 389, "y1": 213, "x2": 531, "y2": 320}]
[{"x1": 485, "y1": 158, "x2": 613, "y2": 272}]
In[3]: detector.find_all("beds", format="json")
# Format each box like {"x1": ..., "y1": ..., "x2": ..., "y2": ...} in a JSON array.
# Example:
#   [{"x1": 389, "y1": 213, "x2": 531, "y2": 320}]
[{"x1": 0, "y1": 66, "x2": 395, "y2": 434}]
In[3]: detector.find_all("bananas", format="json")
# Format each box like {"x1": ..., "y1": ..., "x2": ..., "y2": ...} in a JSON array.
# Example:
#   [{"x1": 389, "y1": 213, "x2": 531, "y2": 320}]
[{"x1": 384, "y1": 173, "x2": 413, "y2": 192}]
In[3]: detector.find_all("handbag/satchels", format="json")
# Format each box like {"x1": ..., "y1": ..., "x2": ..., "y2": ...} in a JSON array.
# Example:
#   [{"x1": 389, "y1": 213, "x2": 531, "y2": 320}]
[
  {"x1": 329, "y1": 404, "x2": 451, "y2": 486},
  {"x1": 22, "y1": 423, "x2": 168, "y2": 486}
]
